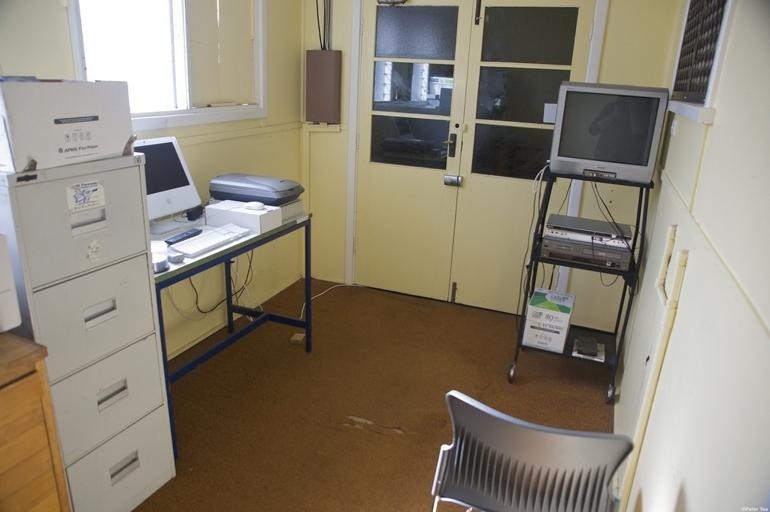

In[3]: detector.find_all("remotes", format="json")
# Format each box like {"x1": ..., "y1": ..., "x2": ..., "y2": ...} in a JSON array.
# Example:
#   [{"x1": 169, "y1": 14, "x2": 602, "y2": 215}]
[{"x1": 165, "y1": 229, "x2": 202, "y2": 245}]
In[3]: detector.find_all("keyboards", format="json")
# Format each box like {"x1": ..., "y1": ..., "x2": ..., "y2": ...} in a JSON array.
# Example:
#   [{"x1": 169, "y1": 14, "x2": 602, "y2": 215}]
[{"x1": 171, "y1": 223, "x2": 251, "y2": 259}]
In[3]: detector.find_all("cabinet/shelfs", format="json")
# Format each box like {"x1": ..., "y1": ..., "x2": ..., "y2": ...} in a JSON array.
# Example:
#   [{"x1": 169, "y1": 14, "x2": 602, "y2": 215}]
[
  {"x1": 508, "y1": 171, "x2": 654, "y2": 406},
  {"x1": 1, "y1": 152, "x2": 178, "y2": 510},
  {"x1": 0, "y1": 333, "x2": 80, "y2": 510}
]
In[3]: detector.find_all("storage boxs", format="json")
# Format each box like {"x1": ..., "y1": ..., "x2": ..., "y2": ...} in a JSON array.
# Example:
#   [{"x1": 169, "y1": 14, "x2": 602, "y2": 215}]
[{"x1": 521, "y1": 287, "x2": 577, "y2": 359}]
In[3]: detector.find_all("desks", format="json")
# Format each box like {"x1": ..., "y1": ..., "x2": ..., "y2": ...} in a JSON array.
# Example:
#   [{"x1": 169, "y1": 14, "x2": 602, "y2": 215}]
[{"x1": 145, "y1": 212, "x2": 321, "y2": 458}]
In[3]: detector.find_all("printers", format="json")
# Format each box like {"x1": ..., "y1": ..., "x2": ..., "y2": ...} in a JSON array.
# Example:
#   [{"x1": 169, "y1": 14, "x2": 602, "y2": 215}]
[{"x1": 209, "y1": 170, "x2": 304, "y2": 206}]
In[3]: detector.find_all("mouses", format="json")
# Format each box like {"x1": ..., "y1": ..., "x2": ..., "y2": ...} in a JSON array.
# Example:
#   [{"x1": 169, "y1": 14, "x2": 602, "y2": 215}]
[{"x1": 245, "y1": 201, "x2": 264, "y2": 210}]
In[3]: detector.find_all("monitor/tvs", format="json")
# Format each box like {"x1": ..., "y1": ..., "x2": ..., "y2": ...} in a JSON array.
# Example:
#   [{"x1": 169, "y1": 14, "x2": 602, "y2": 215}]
[
  {"x1": 133, "y1": 136, "x2": 201, "y2": 235},
  {"x1": 549, "y1": 81, "x2": 669, "y2": 185}
]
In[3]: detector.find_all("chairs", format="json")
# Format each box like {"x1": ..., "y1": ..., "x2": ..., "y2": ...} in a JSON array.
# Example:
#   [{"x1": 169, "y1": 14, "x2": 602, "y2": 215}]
[{"x1": 428, "y1": 390, "x2": 634, "y2": 510}]
